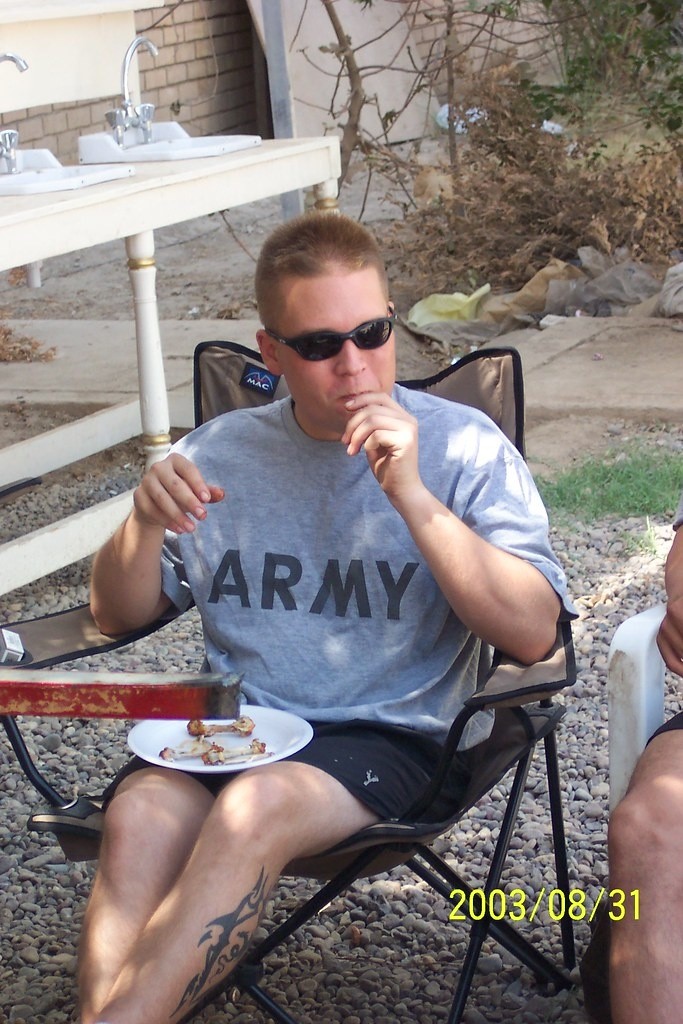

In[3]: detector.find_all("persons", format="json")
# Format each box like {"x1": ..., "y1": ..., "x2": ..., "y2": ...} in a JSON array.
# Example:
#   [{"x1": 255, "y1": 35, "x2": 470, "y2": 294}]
[
  {"x1": 78, "y1": 213, "x2": 580, "y2": 1024},
  {"x1": 607, "y1": 494, "x2": 683, "y2": 1024}
]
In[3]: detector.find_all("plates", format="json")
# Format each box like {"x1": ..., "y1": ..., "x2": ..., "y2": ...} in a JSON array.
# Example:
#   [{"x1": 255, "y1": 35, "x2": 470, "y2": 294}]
[{"x1": 130, "y1": 704, "x2": 313, "y2": 773}]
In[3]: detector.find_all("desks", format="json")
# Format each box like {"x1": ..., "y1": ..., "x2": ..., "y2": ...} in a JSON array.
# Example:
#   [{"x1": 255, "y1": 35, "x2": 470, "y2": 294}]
[{"x1": 0, "y1": 136, "x2": 343, "y2": 592}]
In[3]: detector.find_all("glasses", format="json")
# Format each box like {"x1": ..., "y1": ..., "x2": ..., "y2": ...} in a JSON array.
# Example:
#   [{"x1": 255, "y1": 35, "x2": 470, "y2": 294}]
[{"x1": 265, "y1": 304, "x2": 398, "y2": 361}]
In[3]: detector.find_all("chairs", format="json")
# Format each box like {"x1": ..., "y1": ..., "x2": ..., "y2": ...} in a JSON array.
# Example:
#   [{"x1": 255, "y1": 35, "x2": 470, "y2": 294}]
[{"x1": 0, "y1": 340, "x2": 580, "y2": 1024}]
